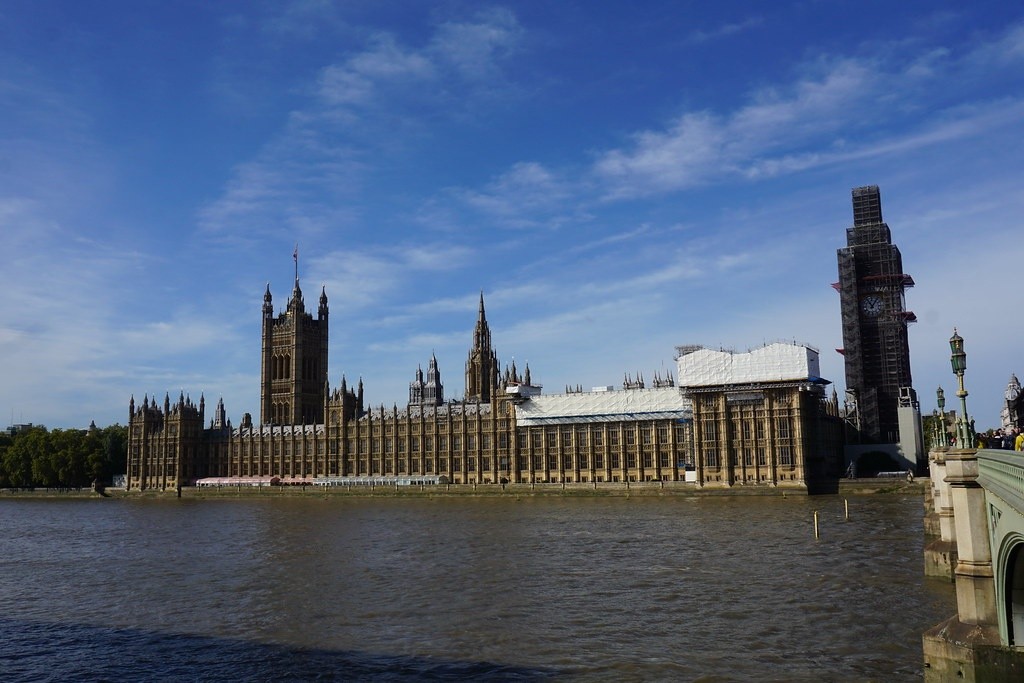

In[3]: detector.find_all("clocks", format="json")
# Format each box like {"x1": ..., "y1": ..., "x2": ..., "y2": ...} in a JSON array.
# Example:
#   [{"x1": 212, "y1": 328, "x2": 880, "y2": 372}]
[{"x1": 860, "y1": 296, "x2": 885, "y2": 318}]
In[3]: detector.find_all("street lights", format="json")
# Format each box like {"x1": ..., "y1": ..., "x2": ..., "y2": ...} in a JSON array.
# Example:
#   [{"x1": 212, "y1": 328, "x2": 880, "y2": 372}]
[
  {"x1": 936, "y1": 384, "x2": 947, "y2": 446},
  {"x1": 933, "y1": 408, "x2": 939, "y2": 447},
  {"x1": 949, "y1": 326, "x2": 971, "y2": 449}
]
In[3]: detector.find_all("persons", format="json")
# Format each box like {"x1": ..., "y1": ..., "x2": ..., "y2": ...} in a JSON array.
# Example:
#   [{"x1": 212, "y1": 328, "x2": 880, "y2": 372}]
[{"x1": 949, "y1": 426, "x2": 1024, "y2": 452}]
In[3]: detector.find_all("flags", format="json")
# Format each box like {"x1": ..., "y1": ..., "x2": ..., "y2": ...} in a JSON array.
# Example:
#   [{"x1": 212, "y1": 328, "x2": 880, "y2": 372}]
[{"x1": 292, "y1": 242, "x2": 298, "y2": 262}]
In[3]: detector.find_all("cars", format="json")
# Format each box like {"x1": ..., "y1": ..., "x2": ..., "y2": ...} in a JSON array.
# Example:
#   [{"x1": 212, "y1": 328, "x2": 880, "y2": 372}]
[
  {"x1": 1004, "y1": 435, "x2": 1015, "y2": 450},
  {"x1": 988, "y1": 436, "x2": 1005, "y2": 448}
]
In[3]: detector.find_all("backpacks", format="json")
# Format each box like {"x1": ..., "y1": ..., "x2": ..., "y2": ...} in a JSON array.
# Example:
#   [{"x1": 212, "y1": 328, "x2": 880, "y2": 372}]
[{"x1": 1019, "y1": 435, "x2": 1024, "y2": 451}]
